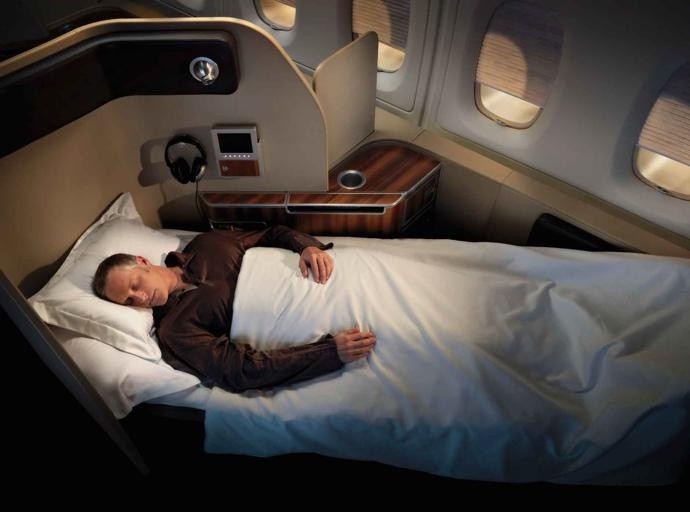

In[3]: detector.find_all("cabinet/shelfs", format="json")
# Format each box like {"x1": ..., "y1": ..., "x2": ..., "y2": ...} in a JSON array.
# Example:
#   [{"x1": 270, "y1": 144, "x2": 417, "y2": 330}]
[{"x1": 198, "y1": 140, "x2": 443, "y2": 236}]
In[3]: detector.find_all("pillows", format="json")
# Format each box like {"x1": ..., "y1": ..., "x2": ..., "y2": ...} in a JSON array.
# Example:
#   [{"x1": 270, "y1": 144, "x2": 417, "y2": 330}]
[{"x1": 27, "y1": 192, "x2": 190, "y2": 362}]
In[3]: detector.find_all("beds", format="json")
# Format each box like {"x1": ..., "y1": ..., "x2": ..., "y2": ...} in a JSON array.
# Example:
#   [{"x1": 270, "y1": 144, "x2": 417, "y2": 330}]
[{"x1": 62, "y1": 226, "x2": 689, "y2": 486}]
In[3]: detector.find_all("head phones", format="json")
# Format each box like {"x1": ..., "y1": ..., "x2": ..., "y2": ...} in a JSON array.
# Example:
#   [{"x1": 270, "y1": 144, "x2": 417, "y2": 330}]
[{"x1": 165, "y1": 133, "x2": 209, "y2": 184}]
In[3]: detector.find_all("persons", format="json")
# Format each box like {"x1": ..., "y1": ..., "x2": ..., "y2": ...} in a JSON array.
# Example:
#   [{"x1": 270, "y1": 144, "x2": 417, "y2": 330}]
[{"x1": 91, "y1": 223, "x2": 376, "y2": 393}]
